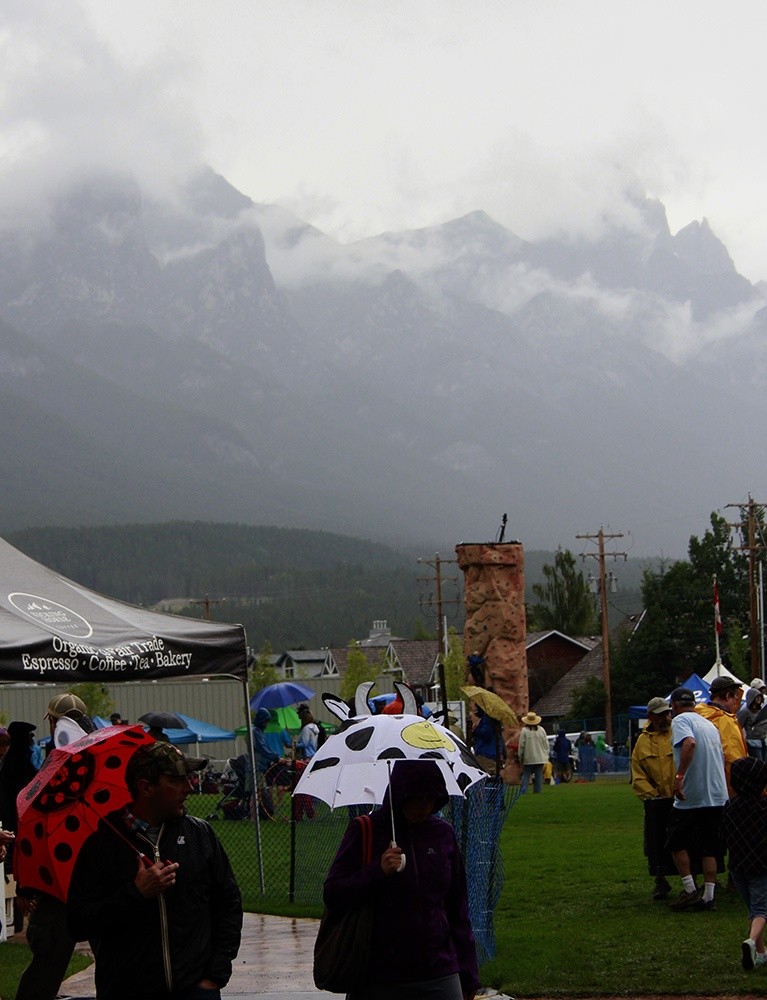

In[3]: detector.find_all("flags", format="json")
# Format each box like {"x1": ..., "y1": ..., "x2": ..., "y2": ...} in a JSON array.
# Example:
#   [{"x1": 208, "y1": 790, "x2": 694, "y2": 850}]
[{"x1": 712, "y1": 579, "x2": 723, "y2": 634}]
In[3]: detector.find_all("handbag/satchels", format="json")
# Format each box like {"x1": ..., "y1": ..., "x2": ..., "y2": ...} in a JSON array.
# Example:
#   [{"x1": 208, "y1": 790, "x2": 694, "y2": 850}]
[{"x1": 313, "y1": 913, "x2": 359, "y2": 993}]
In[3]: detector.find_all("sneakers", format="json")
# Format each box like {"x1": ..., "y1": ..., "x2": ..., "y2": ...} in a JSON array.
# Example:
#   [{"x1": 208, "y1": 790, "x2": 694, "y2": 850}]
[
  {"x1": 754, "y1": 945, "x2": 767, "y2": 964},
  {"x1": 741, "y1": 939, "x2": 755, "y2": 970}
]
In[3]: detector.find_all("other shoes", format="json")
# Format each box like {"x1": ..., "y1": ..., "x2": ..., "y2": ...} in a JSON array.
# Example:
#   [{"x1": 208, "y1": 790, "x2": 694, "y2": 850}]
[
  {"x1": 670, "y1": 889, "x2": 715, "y2": 913},
  {"x1": 653, "y1": 889, "x2": 668, "y2": 899},
  {"x1": 703, "y1": 881, "x2": 720, "y2": 891}
]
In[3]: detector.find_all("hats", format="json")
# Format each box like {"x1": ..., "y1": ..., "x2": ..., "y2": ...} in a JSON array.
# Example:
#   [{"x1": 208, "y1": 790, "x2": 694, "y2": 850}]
[
  {"x1": 44, "y1": 693, "x2": 88, "y2": 721},
  {"x1": 709, "y1": 677, "x2": 743, "y2": 693},
  {"x1": 729, "y1": 758, "x2": 767, "y2": 796},
  {"x1": 668, "y1": 689, "x2": 695, "y2": 708},
  {"x1": 521, "y1": 712, "x2": 541, "y2": 725},
  {"x1": 125, "y1": 741, "x2": 208, "y2": 784},
  {"x1": 750, "y1": 678, "x2": 766, "y2": 689},
  {"x1": 8, "y1": 721, "x2": 36, "y2": 736},
  {"x1": 647, "y1": 697, "x2": 673, "y2": 714}
]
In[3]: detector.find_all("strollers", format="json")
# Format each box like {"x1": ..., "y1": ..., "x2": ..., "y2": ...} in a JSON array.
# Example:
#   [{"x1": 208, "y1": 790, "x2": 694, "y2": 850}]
[{"x1": 205, "y1": 753, "x2": 262, "y2": 821}]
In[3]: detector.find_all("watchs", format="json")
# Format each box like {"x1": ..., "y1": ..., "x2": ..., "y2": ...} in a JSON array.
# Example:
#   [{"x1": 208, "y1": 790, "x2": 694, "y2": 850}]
[{"x1": 675, "y1": 773, "x2": 684, "y2": 780}]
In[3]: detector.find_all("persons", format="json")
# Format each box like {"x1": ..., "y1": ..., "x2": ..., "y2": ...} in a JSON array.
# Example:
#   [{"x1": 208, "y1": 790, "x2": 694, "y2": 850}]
[
  {"x1": 553, "y1": 730, "x2": 572, "y2": 785},
  {"x1": 0, "y1": 691, "x2": 245, "y2": 1000},
  {"x1": 322, "y1": 761, "x2": 474, "y2": 1000},
  {"x1": 573, "y1": 677, "x2": 767, "y2": 970},
  {"x1": 243, "y1": 682, "x2": 485, "y2": 1000},
  {"x1": 472, "y1": 703, "x2": 507, "y2": 786},
  {"x1": 517, "y1": 711, "x2": 550, "y2": 793}
]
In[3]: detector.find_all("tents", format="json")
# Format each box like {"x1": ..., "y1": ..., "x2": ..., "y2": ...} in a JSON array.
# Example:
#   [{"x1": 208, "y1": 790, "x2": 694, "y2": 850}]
[
  {"x1": 0, "y1": 536, "x2": 266, "y2": 1000},
  {"x1": 136, "y1": 712, "x2": 236, "y2": 794},
  {"x1": 628, "y1": 659, "x2": 767, "y2": 784}
]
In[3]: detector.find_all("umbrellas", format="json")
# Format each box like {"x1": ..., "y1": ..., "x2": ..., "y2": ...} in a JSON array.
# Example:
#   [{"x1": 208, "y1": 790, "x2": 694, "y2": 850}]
[
  {"x1": 247, "y1": 683, "x2": 316, "y2": 736},
  {"x1": 289, "y1": 680, "x2": 492, "y2": 871},
  {"x1": 11, "y1": 724, "x2": 175, "y2": 903},
  {"x1": 460, "y1": 685, "x2": 519, "y2": 728},
  {"x1": 32, "y1": 716, "x2": 118, "y2": 748},
  {"x1": 236, "y1": 706, "x2": 337, "y2": 743}
]
